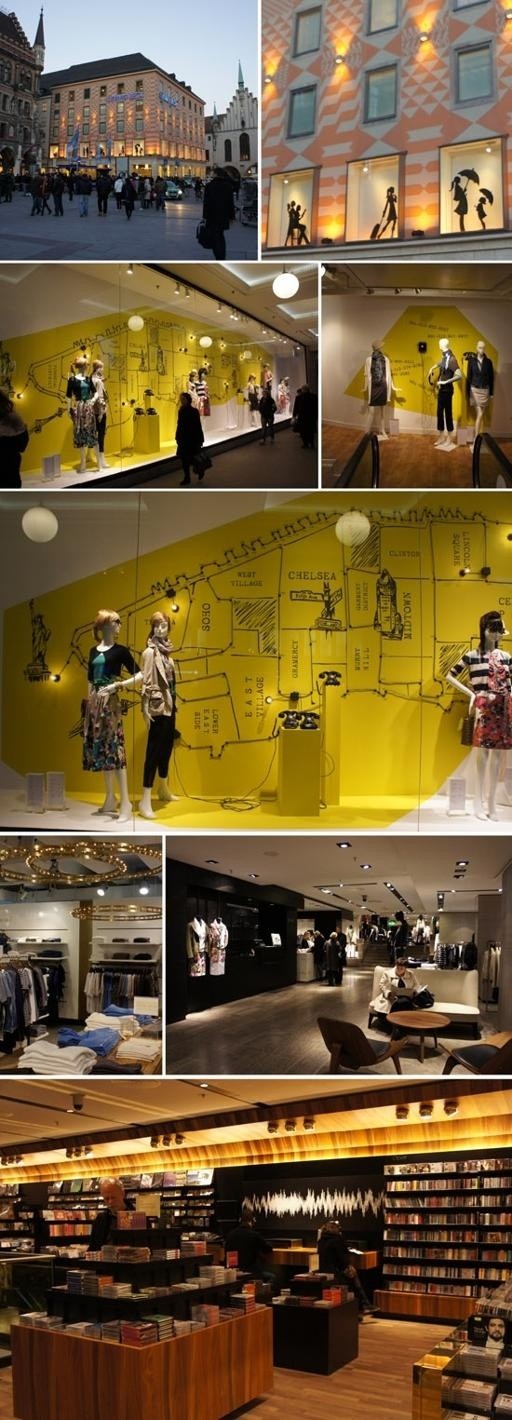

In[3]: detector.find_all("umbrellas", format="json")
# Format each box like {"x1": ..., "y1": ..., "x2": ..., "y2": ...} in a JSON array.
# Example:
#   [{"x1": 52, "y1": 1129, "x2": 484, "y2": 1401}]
[{"x1": 458, "y1": 168, "x2": 479, "y2": 188}]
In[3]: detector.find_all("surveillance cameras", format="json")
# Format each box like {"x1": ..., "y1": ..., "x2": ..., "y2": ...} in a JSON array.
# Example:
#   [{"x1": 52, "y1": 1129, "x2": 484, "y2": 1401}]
[
  {"x1": 73, "y1": 1095, "x2": 83, "y2": 1110},
  {"x1": 363, "y1": 895, "x2": 366, "y2": 901}
]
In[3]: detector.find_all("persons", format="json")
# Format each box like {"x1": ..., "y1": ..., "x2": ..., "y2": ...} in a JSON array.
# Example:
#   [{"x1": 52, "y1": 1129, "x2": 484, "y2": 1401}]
[
  {"x1": 195, "y1": 367, "x2": 209, "y2": 417},
  {"x1": 87, "y1": 360, "x2": 112, "y2": 470},
  {"x1": 80, "y1": 607, "x2": 144, "y2": 823},
  {"x1": 0, "y1": 388, "x2": 29, "y2": 486},
  {"x1": 284, "y1": 176, "x2": 493, "y2": 247},
  {"x1": 66, "y1": 358, "x2": 99, "y2": 472},
  {"x1": 185, "y1": 913, "x2": 208, "y2": 978},
  {"x1": 445, "y1": 609, "x2": 512, "y2": 820},
  {"x1": 237, "y1": 362, "x2": 315, "y2": 449},
  {"x1": 427, "y1": 339, "x2": 462, "y2": 446},
  {"x1": 175, "y1": 392, "x2": 205, "y2": 486},
  {"x1": 187, "y1": 369, "x2": 200, "y2": 408},
  {"x1": 136, "y1": 612, "x2": 179, "y2": 813},
  {"x1": 465, "y1": 341, "x2": 495, "y2": 438},
  {"x1": 300, "y1": 910, "x2": 430, "y2": 1035},
  {"x1": 0, "y1": 163, "x2": 236, "y2": 260},
  {"x1": 206, "y1": 918, "x2": 231, "y2": 976},
  {"x1": 361, "y1": 341, "x2": 401, "y2": 442}
]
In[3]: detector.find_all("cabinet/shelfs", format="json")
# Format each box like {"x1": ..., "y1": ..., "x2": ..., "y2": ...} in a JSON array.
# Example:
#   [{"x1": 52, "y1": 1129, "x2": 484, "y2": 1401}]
[
  {"x1": 266, "y1": 1298, "x2": 358, "y2": 1375},
  {"x1": 6, "y1": 942, "x2": 68, "y2": 960},
  {"x1": 379, "y1": 1171, "x2": 481, "y2": 1298},
  {"x1": 45, "y1": 1185, "x2": 217, "y2": 1231},
  {"x1": 478, "y1": 1169, "x2": 512, "y2": 1298},
  {"x1": 88, "y1": 942, "x2": 162, "y2": 963},
  {"x1": 11, "y1": 1307, "x2": 273, "y2": 1420}
]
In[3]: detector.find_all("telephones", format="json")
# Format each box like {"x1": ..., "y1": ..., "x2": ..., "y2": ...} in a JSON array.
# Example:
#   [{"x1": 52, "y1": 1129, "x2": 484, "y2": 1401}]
[
  {"x1": 135, "y1": 407, "x2": 145, "y2": 414},
  {"x1": 144, "y1": 389, "x2": 155, "y2": 396},
  {"x1": 319, "y1": 671, "x2": 341, "y2": 686},
  {"x1": 278, "y1": 710, "x2": 321, "y2": 729},
  {"x1": 147, "y1": 407, "x2": 157, "y2": 414}
]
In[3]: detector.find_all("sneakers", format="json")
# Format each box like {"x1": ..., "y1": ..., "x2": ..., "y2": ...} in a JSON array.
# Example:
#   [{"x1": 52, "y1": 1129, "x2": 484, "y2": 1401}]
[
  {"x1": 363, "y1": 1303, "x2": 381, "y2": 1314},
  {"x1": 30, "y1": 210, "x2": 64, "y2": 217},
  {"x1": 79, "y1": 203, "x2": 167, "y2": 221},
  {"x1": 336, "y1": 983, "x2": 342, "y2": 986},
  {"x1": 197, "y1": 468, "x2": 205, "y2": 480},
  {"x1": 180, "y1": 475, "x2": 190, "y2": 486},
  {"x1": 326, "y1": 983, "x2": 334, "y2": 986}
]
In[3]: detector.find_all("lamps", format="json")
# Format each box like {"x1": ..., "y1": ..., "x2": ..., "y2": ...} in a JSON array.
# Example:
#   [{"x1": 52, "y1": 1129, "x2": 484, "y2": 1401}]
[
  {"x1": 335, "y1": 55, "x2": 343, "y2": 63},
  {"x1": 128, "y1": 313, "x2": 144, "y2": 331},
  {"x1": 66, "y1": 1146, "x2": 93, "y2": 1158},
  {"x1": 150, "y1": 1133, "x2": 185, "y2": 1148},
  {"x1": 22, "y1": 497, "x2": 58, "y2": 543},
  {"x1": 486, "y1": 142, "x2": 496, "y2": 152},
  {"x1": 396, "y1": 1101, "x2": 458, "y2": 1121},
  {"x1": 97, "y1": 883, "x2": 109, "y2": 896},
  {"x1": 125, "y1": 264, "x2": 301, "y2": 351},
  {"x1": 283, "y1": 175, "x2": 289, "y2": 183},
  {"x1": 321, "y1": 238, "x2": 333, "y2": 244},
  {"x1": 268, "y1": 1117, "x2": 315, "y2": 1135},
  {"x1": 139, "y1": 880, "x2": 149, "y2": 895},
  {"x1": 363, "y1": 163, "x2": 368, "y2": 172},
  {"x1": 244, "y1": 350, "x2": 252, "y2": 359},
  {"x1": 272, "y1": 265, "x2": 299, "y2": 300},
  {"x1": 200, "y1": 336, "x2": 212, "y2": 348},
  {"x1": 506, "y1": 9, "x2": 512, "y2": 19},
  {"x1": 412, "y1": 230, "x2": 424, "y2": 236},
  {"x1": 335, "y1": 508, "x2": 370, "y2": 547},
  {"x1": 419, "y1": 32, "x2": 429, "y2": 42},
  {"x1": 264, "y1": 76, "x2": 271, "y2": 83},
  {"x1": 1, "y1": 1155, "x2": 24, "y2": 1166}
]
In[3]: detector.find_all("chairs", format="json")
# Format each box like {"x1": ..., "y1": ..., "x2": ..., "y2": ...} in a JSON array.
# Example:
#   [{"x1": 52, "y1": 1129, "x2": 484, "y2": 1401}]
[
  {"x1": 317, "y1": 1018, "x2": 408, "y2": 1074},
  {"x1": 439, "y1": 1031, "x2": 512, "y2": 1074}
]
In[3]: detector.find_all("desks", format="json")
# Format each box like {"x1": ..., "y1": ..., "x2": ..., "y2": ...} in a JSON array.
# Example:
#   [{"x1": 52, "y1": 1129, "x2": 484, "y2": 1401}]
[
  {"x1": 272, "y1": 1247, "x2": 383, "y2": 1272},
  {"x1": 386, "y1": 1011, "x2": 451, "y2": 1059}
]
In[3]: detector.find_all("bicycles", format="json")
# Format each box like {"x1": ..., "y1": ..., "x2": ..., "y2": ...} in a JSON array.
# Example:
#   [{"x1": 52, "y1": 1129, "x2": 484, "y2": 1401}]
[{"x1": 196, "y1": 185, "x2": 204, "y2": 202}]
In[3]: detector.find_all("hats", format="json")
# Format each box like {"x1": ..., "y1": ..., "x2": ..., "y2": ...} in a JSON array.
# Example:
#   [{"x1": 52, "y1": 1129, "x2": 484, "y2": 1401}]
[{"x1": 372, "y1": 339, "x2": 385, "y2": 350}]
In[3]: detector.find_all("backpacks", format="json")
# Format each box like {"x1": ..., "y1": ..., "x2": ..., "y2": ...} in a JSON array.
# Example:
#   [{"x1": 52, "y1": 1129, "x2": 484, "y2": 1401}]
[{"x1": 99, "y1": 176, "x2": 112, "y2": 195}]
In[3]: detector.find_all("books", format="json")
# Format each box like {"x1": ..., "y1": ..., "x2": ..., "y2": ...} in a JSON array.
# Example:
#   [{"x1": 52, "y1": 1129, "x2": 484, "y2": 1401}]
[{"x1": 0, "y1": 1160, "x2": 511, "y2": 1420}]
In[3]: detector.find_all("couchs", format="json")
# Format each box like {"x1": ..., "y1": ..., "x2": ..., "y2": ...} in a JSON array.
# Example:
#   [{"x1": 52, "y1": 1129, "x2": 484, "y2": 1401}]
[{"x1": 368, "y1": 965, "x2": 481, "y2": 1040}]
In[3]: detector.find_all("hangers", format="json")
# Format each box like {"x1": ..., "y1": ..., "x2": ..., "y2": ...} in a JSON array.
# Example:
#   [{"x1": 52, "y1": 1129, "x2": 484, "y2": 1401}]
[
  {"x1": 89, "y1": 962, "x2": 154, "y2": 976},
  {"x1": 0, "y1": 955, "x2": 33, "y2": 970}
]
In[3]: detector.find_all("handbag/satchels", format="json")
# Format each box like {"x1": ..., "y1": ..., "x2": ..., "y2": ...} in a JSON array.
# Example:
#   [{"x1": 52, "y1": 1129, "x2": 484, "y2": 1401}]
[
  {"x1": 192, "y1": 450, "x2": 213, "y2": 474},
  {"x1": 321, "y1": 950, "x2": 328, "y2": 970},
  {"x1": 414, "y1": 988, "x2": 434, "y2": 1009},
  {"x1": 196, "y1": 219, "x2": 212, "y2": 250},
  {"x1": 461, "y1": 713, "x2": 476, "y2": 747},
  {"x1": 81, "y1": 180, "x2": 92, "y2": 193}
]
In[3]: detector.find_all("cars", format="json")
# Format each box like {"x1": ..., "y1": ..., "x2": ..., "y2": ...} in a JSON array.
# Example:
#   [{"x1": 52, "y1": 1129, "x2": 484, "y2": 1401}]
[{"x1": 165, "y1": 177, "x2": 192, "y2": 200}]
